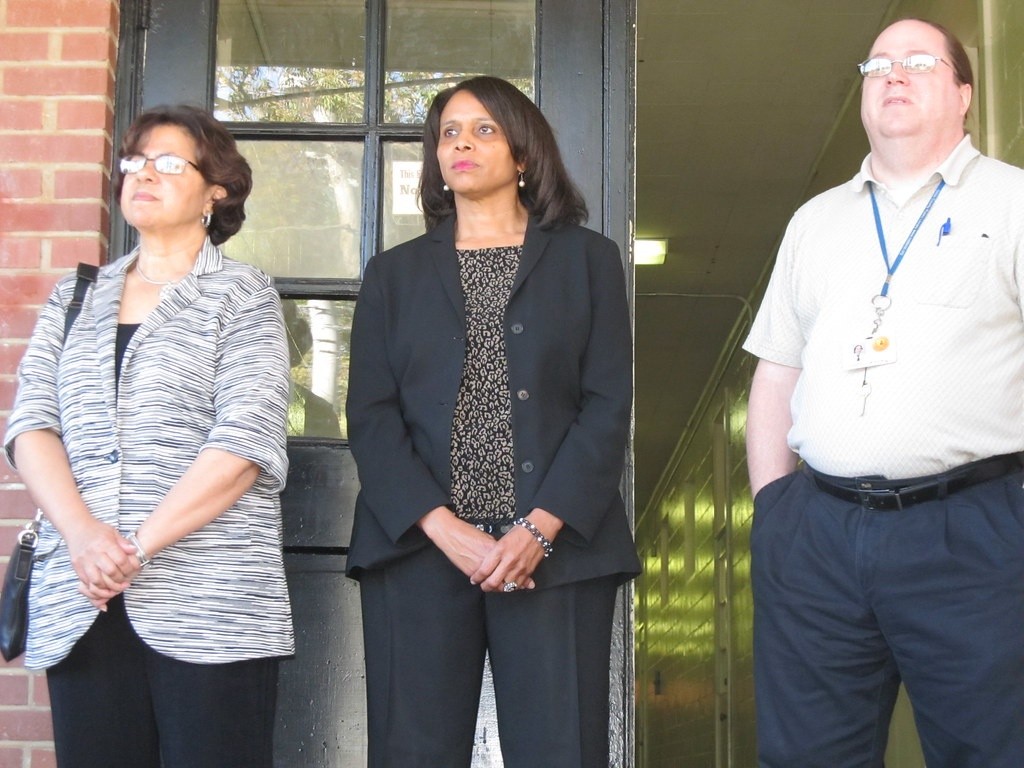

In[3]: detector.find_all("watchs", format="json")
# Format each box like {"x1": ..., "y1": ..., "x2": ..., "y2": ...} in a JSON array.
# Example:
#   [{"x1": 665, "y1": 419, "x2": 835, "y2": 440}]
[{"x1": 124, "y1": 531, "x2": 152, "y2": 573}]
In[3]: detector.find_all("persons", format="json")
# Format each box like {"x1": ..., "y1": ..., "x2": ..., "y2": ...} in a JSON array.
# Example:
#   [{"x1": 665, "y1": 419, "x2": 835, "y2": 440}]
[
  {"x1": 744, "y1": 16, "x2": 1024, "y2": 768},
  {"x1": 1, "y1": 100, "x2": 302, "y2": 768},
  {"x1": 339, "y1": 74, "x2": 645, "y2": 766}
]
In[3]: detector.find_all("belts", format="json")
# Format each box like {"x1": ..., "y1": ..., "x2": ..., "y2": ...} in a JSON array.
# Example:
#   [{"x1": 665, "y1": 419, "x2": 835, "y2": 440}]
[{"x1": 801, "y1": 453, "x2": 1024, "y2": 511}]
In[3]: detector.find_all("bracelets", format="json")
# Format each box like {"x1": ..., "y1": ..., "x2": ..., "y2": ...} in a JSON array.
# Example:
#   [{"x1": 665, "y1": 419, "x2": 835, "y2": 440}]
[{"x1": 513, "y1": 516, "x2": 554, "y2": 559}]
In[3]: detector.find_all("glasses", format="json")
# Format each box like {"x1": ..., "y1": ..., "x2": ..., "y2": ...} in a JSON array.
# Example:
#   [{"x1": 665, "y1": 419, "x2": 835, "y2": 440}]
[
  {"x1": 118, "y1": 152, "x2": 200, "y2": 175},
  {"x1": 857, "y1": 54, "x2": 964, "y2": 84}
]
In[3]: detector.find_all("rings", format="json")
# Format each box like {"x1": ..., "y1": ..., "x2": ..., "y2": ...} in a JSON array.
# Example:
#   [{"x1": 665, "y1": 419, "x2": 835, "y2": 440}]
[{"x1": 501, "y1": 579, "x2": 519, "y2": 593}]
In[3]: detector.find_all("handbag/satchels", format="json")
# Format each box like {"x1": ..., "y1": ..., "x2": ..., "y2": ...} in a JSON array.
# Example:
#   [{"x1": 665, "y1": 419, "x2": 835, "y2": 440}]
[{"x1": 0, "y1": 519, "x2": 40, "y2": 663}]
